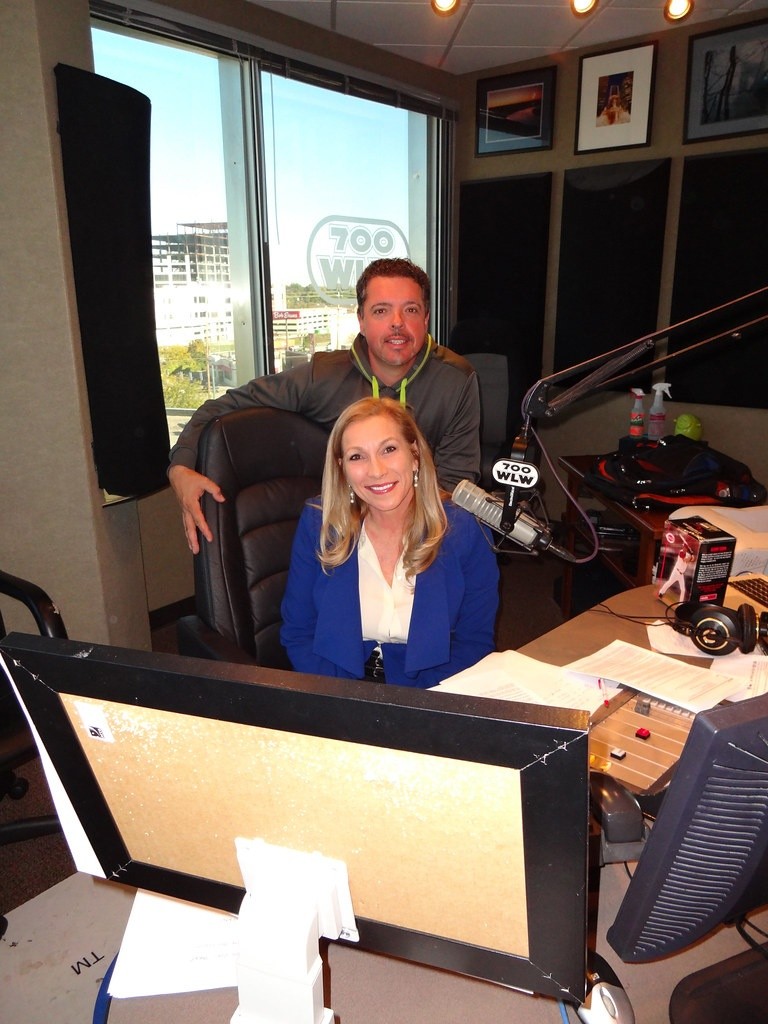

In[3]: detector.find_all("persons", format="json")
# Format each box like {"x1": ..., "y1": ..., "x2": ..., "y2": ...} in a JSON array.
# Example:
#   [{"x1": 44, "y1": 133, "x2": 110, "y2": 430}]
[
  {"x1": 659, "y1": 542, "x2": 694, "y2": 606},
  {"x1": 285, "y1": 397, "x2": 503, "y2": 689},
  {"x1": 168, "y1": 257, "x2": 483, "y2": 555}
]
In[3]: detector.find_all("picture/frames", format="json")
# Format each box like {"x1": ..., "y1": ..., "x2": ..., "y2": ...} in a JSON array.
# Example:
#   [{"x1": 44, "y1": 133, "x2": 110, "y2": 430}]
[
  {"x1": 473, "y1": 65, "x2": 557, "y2": 157},
  {"x1": 682, "y1": 16, "x2": 768, "y2": 146},
  {"x1": 573, "y1": 39, "x2": 659, "y2": 154}
]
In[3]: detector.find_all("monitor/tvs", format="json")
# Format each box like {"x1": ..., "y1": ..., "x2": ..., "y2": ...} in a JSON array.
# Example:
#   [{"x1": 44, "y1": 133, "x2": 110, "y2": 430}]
[
  {"x1": 1, "y1": 631, "x2": 589, "y2": 1006},
  {"x1": 607, "y1": 693, "x2": 768, "y2": 964}
]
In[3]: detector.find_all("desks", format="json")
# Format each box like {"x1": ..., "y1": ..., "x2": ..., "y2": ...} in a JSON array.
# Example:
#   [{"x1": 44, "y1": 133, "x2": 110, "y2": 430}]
[
  {"x1": 559, "y1": 455, "x2": 671, "y2": 622},
  {"x1": 2, "y1": 581, "x2": 768, "y2": 1024}
]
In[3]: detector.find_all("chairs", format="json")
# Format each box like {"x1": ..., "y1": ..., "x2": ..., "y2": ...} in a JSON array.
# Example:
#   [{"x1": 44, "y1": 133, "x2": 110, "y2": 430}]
[{"x1": 0, "y1": 570, "x2": 69, "y2": 847}]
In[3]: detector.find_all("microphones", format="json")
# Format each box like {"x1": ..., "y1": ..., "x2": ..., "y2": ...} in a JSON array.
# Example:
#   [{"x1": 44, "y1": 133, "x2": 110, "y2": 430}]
[{"x1": 451, "y1": 479, "x2": 578, "y2": 566}]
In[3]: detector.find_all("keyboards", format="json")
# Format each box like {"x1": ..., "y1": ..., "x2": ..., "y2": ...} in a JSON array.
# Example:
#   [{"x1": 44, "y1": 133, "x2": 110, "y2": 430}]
[{"x1": 727, "y1": 577, "x2": 768, "y2": 610}]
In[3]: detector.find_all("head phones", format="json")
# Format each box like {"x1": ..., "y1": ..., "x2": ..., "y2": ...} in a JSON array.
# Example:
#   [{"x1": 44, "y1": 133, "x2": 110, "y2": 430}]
[{"x1": 674, "y1": 601, "x2": 759, "y2": 657}]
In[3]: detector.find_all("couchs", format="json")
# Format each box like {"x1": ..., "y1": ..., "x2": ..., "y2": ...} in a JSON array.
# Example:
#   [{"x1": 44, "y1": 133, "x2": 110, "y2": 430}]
[{"x1": 178, "y1": 404, "x2": 327, "y2": 674}]
